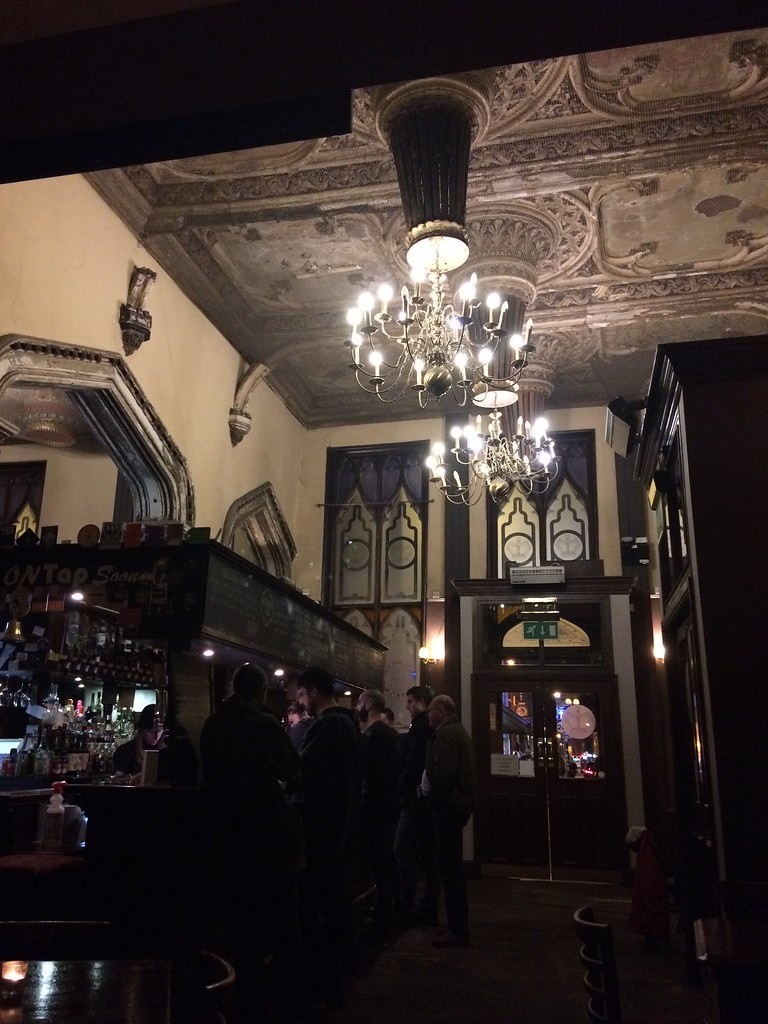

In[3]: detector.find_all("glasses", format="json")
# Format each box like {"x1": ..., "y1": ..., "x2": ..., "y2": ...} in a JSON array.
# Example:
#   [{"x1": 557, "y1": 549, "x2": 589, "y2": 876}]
[{"x1": 427, "y1": 707, "x2": 437, "y2": 713}]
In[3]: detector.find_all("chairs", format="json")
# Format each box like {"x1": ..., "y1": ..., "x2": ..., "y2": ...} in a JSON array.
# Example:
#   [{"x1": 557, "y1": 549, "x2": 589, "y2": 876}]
[
  {"x1": 0, "y1": 920, "x2": 114, "y2": 960},
  {"x1": 573, "y1": 905, "x2": 622, "y2": 1024},
  {"x1": 195, "y1": 950, "x2": 237, "y2": 1024}
]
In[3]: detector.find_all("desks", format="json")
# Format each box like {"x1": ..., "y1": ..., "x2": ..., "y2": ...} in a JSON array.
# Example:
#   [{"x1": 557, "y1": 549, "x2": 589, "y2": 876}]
[
  {"x1": 694, "y1": 918, "x2": 768, "y2": 1024},
  {"x1": 0, "y1": 958, "x2": 172, "y2": 1024}
]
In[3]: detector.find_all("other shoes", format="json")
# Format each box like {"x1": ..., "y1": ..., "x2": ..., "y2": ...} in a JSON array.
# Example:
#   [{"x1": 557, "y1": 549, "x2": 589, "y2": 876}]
[
  {"x1": 411, "y1": 911, "x2": 439, "y2": 927},
  {"x1": 431, "y1": 931, "x2": 468, "y2": 946}
]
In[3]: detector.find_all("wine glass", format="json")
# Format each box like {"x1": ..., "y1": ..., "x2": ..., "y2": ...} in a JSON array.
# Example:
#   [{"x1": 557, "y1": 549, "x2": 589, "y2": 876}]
[{"x1": 0, "y1": 674, "x2": 30, "y2": 707}]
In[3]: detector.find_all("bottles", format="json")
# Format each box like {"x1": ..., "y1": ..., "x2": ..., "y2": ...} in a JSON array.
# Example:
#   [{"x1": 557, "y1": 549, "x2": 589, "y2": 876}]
[
  {"x1": 42, "y1": 780, "x2": 66, "y2": 850},
  {"x1": 8, "y1": 684, "x2": 134, "y2": 775}
]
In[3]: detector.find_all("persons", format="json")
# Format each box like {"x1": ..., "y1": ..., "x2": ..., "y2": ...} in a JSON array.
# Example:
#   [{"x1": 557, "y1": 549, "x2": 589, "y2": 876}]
[
  {"x1": 113, "y1": 705, "x2": 162, "y2": 775},
  {"x1": 417, "y1": 694, "x2": 476, "y2": 948},
  {"x1": 199, "y1": 665, "x2": 302, "y2": 968},
  {"x1": 356, "y1": 685, "x2": 439, "y2": 932},
  {"x1": 288, "y1": 669, "x2": 361, "y2": 1007}
]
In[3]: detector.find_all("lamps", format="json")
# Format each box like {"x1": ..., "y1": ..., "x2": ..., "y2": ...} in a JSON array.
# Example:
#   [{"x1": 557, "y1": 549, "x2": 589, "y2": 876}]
[
  {"x1": 423, "y1": 294, "x2": 563, "y2": 506},
  {"x1": 418, "y1": 647, "x2": 437, "y2": 664},
  {"x1": 653, "y1": 646, "x2": 665, "y2": 663},
  {"x1": 344, "y1": 77, "x2": 536, "y2": 410}
]
